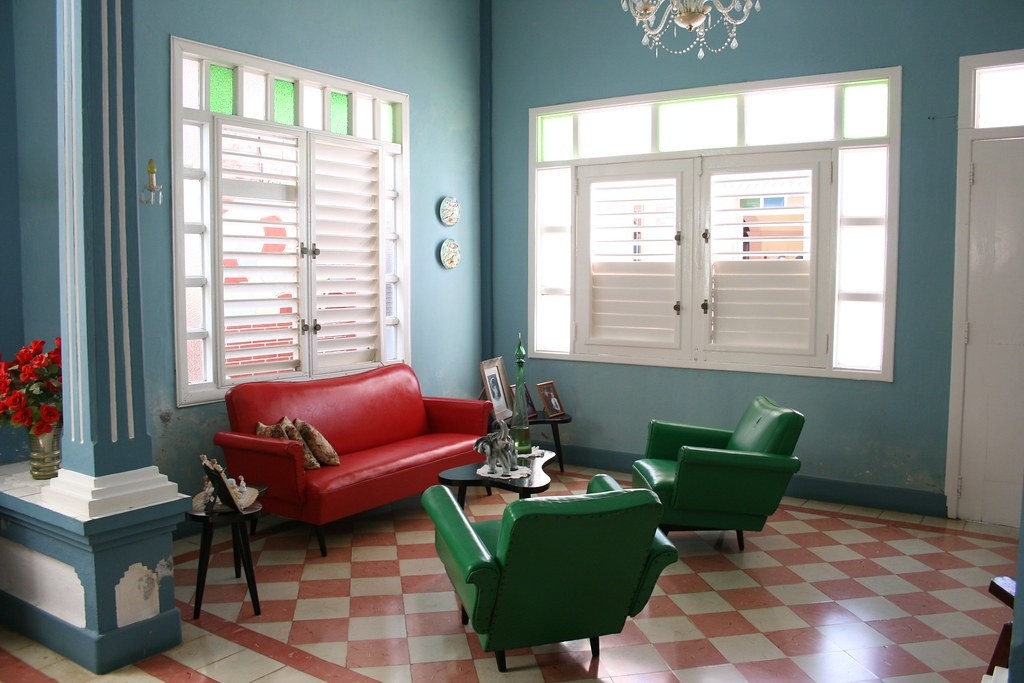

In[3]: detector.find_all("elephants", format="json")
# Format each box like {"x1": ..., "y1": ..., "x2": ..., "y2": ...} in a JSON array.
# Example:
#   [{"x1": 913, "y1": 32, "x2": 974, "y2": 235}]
[{"x1": 472, "y1": 419, "x2": 519, "y2": 477}]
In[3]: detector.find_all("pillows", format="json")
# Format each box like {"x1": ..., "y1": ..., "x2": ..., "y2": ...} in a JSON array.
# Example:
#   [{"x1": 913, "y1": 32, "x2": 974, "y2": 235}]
[
  {"x1": 255, "y1": 420, "x2": 289, "y2": 439},
  {"x1": 295, "y1": 417, "x2": 342, "y2": 467},
  {"x1": 281, "y1": 415, "x2": 322, "y2": 469}
]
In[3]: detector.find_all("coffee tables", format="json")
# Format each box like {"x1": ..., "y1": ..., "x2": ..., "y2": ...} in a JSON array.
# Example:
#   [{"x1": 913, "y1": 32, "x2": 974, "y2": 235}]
[{"x1": 437, "y1": 448, "x2": 558, "y2": 513}]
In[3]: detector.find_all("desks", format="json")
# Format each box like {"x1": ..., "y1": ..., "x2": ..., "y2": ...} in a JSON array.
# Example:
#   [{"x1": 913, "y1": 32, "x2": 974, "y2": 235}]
[
  {"x1": 489, "y1": 410, "x2": 572, "y2": 475},
  {"x1": 186, "y1": 479, "x2": 269, "y2": 620}
]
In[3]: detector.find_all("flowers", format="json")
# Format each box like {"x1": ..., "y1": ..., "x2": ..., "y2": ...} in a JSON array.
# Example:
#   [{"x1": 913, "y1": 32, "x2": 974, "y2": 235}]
[{"x1": 0, "y1": 337, "x2": 64, "y2": 437}]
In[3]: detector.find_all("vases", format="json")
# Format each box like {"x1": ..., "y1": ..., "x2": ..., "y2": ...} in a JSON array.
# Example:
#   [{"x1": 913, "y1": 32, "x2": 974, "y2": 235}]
[{"x1": 25, "y1": 412, "x2": 63, "y2": 479}]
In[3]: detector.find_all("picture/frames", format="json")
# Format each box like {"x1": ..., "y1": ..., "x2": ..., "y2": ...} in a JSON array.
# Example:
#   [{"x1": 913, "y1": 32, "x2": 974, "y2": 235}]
[
  {"x1": 537, "y1": 381, "x2": 566, "y2": 418},
  {"x1": 479, "y1": 356, "x2": 514, "y2": 421},
  {"x1": 508, "y1": 382, "x2": 538, "y2": 418}
]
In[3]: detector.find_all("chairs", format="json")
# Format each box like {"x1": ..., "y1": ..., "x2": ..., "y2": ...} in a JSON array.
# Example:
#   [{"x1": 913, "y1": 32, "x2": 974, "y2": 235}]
[
  {"x1": 417, "y1": 472, "x2": 676, "y2": 675},
  {"x1": 630, "y1": 397, "x2": 805, "y2": 552}
]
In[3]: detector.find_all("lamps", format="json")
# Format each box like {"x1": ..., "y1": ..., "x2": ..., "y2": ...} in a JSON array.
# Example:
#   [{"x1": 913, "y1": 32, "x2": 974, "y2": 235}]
[
  {"x1": 141, "y1": 158, "x2": 165, "y2": 206},
  {"x1": 620, "y1": 0, "x2": 762, "y2": 62}
]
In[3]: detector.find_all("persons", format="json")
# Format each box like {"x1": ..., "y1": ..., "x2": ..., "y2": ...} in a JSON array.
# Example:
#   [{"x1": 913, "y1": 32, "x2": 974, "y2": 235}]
[
  {"x1": 199, "y1": 455, "x2": 246, "y2": 503},
  {"x1": 545, "y1": 391, "x2": 560, "y2": 414},
  {"x1": 489, "y1": 378, "x2": 502, "y2": 406}
]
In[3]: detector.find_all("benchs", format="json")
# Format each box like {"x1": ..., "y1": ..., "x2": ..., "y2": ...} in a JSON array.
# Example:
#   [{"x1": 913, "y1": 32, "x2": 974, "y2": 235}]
[{"x1": 212, "y1": 363, "x2": 493, "y2": 557}]
